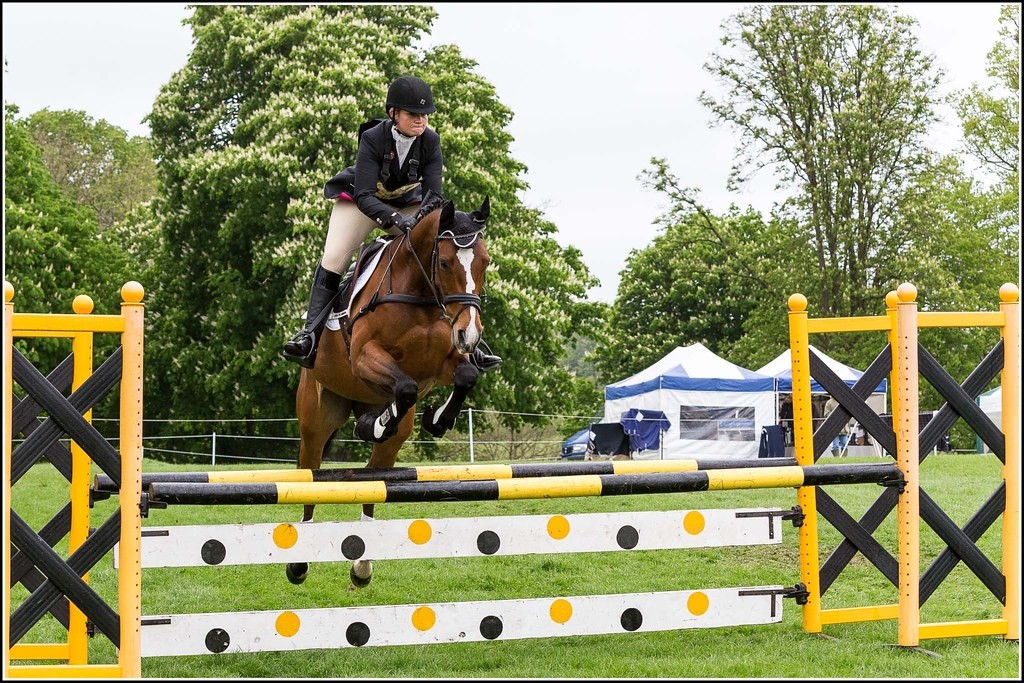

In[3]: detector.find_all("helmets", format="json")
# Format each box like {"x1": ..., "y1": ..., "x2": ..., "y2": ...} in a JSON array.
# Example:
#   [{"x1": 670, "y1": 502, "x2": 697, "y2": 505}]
[{"x1": 386, "y1": 76, "x2": 437, "y2": 114}]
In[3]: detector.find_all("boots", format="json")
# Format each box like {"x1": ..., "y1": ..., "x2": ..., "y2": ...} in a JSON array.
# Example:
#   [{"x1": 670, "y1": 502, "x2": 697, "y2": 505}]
[
  {"x1": 831, "y1": 449, "x2": 839, "y2": 457},
  {"x1": 841, "y1": 449, "x2": 848, "y2": 458},
  {"x1": 851, "y1": 432, "x2": 860, "y2": 446},
  {"x1": 469, "y1": 345, "x2": 503, "y2": 369},
  {"x1": 284, "y1": 263, "x2": 343, "y2": 370},
  {"x1": 863, "y1": 435, "x2": 872, "y2": 446}
]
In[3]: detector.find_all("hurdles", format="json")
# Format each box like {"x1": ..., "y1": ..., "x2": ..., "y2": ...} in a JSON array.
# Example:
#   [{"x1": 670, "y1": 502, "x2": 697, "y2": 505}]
[{"x1": 4, "y1": 279, "x2": 1019, "y2": 680}]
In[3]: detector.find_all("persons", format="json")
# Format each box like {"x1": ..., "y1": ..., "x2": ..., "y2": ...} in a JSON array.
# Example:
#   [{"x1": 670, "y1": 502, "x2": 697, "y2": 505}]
[
  {"x1": 779, "y1": 393, "x2": 820, "y2": 442},
  {"x1": 824, "y1": 395, "x2": 850, "y2": 458},
  {"x1": 284, "y1": 77, "x2": 503, "y2": 369}
]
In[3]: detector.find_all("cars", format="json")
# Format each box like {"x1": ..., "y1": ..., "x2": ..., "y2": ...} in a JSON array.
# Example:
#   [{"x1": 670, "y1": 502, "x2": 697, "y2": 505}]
[{"x1": 560, "y1": 428, "x2": 590, "y2": 460}]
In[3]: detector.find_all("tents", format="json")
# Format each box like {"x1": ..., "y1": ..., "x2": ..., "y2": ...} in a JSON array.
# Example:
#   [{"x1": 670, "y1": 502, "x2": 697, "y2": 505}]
[
  {"x1": 604, "y1": 342, "x2": 778, "y2": 461},
  {"x1": 754, "y1": 344, "x2": 887, "y2": 458}
]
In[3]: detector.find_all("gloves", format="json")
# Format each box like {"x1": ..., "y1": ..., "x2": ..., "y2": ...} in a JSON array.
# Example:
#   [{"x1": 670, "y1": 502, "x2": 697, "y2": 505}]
[{"x1": 391, "y1": 211, "x2": 419, "y2": 233}]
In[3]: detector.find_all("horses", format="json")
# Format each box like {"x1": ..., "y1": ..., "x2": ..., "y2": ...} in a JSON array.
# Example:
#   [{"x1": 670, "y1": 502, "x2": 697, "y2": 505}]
[{"x1": 284, "y1": 194, "x2": 491, "y2": 589}]
